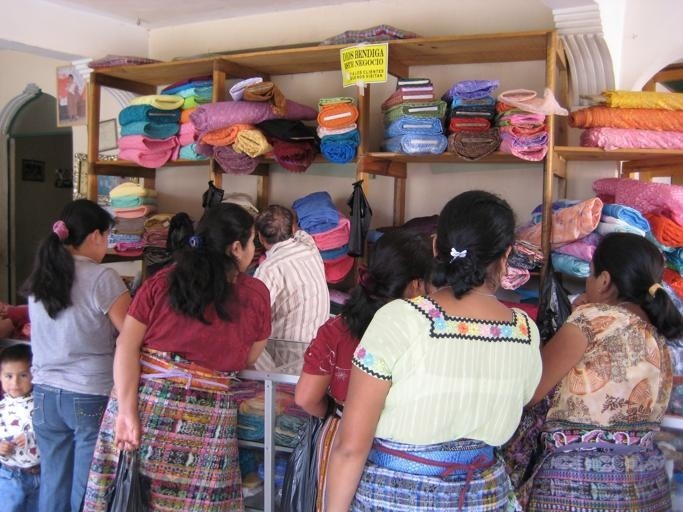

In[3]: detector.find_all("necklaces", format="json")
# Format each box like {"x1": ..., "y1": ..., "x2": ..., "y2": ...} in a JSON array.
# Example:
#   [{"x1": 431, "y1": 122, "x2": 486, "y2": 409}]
[
  {"x1": 432, "y1": 284, "x2": 497, "y2": 299},
  {"x1": 617, "y1": 301, "x2": 636, "y2": 305}
]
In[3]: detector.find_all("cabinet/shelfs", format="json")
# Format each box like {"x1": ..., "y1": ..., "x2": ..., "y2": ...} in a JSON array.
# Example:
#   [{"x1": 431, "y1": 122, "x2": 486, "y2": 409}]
[
  {"x1": 544, "y1": 65, "x2": 682, "y2": 300},
  {"x1": 87, "y1": 31, "x2": 568, "y2": 317},
  {"x1": 0, "y1": 303, "x2": 683, "y2": 512}
]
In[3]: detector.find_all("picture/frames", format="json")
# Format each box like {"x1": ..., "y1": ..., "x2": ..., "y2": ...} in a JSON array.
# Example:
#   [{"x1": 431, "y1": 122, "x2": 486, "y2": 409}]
[
  {"x1": 56, "y1": 58, "x2": 95, "y2": 128},
  {"x1": 98, "y1": 119, "x2": 118, "y2": 152},
  {"x1": 71, "y1": 152, "x2": 140, "y2": 204}
]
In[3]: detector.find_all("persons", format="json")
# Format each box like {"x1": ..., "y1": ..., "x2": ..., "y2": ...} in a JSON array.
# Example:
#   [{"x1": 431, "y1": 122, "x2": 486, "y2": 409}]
[
  {"x1": 84, "y1": 202, "x2": 272, "y2": 511},
  {"x1": 253, "y1": 205, "x2": 331, "y2": 371},
  {"x1": 282, "y1": 231, "x2": 434, "y2": 511},
  {"x1": 525, "y1": 233, "x2": 682, "y2": 511},
  {"x1": 1, "y1": 344, "x2": 41, "y2": 512},
  {"x1": 18, "y1": 199, "x2": 132, "y2": 510},
  {"x1": 324, "y1": 189, "x2": 545, "y2": 511}
]
